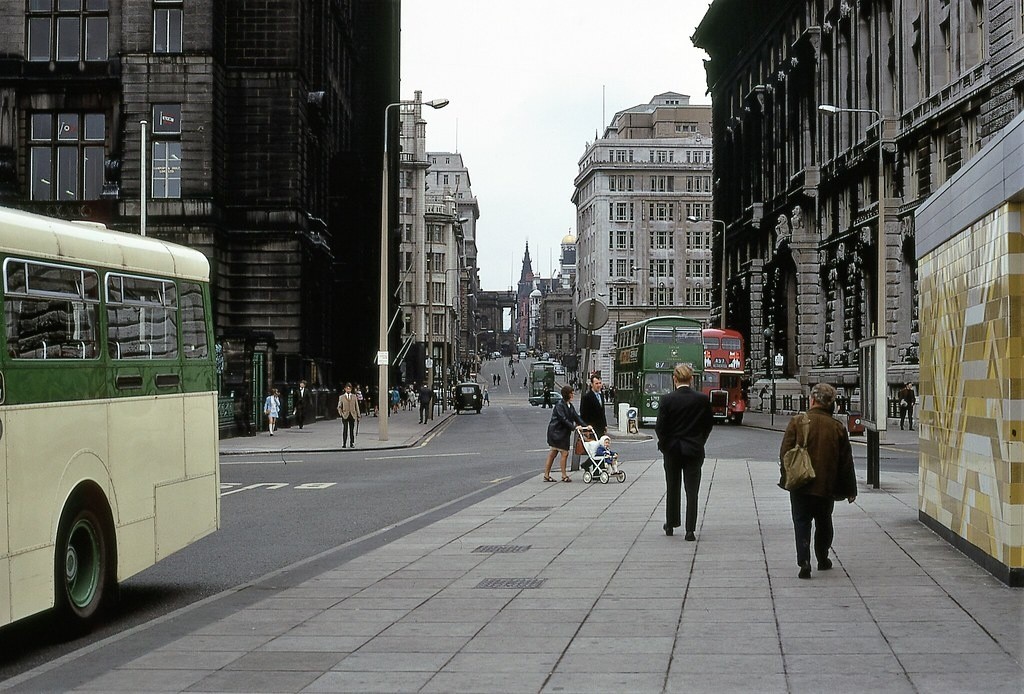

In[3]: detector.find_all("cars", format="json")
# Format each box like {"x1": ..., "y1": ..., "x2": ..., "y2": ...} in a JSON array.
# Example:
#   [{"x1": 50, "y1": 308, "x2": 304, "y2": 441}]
[
  {"x1": 528, "y1": 392, "x2": 563, "y2": 406},
  {"x1": 511, "y1": 352, "x2": 565, "y2": 375},
  {"x1": 489, "y1": 351, "x2": 501, "y2": 359},
  {"x1": 433, "y1": 383, "x2": 483, "y2": 414}
]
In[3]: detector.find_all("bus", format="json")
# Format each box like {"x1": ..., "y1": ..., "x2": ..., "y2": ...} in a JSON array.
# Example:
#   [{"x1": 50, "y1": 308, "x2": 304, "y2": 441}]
[
  {"x1": 0, "y1": 207, "x2": 220, "y2": 636},
  {"x1": 698, "y1": 329, "x2": 745, "y2": 425},
  {"x1": 613, "y1": 316, "x2": 705, "y2": 427},
  {"x1": 529, "y1": 361, "x2": 555, "y2": 402}
]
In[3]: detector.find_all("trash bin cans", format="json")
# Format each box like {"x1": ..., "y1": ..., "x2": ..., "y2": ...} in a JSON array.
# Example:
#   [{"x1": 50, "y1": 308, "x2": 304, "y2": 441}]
[{"x1": 846, "y1": 410, "x2": 865, "y2": 436}]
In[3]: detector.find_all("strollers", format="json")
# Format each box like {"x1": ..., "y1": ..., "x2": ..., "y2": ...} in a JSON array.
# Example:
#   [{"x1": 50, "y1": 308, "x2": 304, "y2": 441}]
[{"x1": 575, "y1": 427, "x2": 626, "y2": 484}]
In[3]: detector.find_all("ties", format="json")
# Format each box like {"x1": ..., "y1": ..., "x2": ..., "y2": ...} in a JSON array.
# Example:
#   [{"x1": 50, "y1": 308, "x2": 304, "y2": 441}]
[
  {"x1": 348, "y1": 394, "x2": 349, "y2": 399},
  {"x1": 596, "y1": 393, "x2": 599, "y2": 398}
]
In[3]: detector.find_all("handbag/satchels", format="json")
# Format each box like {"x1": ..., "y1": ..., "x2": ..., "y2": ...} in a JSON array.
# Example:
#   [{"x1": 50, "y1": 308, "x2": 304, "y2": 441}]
[
  {"x1": 266, "y1": 409, "x2": 271, "y2": 414},
  {"x1": 576, "y1": 434, "x2": 595, "y2": 455},
  {"x1": 784, "y1": 414, "x2": 815, "y2": 490}
]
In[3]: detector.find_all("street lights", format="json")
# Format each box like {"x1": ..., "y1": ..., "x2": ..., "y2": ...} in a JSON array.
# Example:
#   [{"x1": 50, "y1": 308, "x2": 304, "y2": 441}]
[
  {"x1": 450, "y1": 293, "x2": 474, "y2": 365},
  {"x1": 428, "y1": 216, "x2": 469, "y2": 420},
  {"x1": 689, "y1": 216, "x2": 726, "y2": 330},
  {"x1": 821, "y1": 105, "x2": 886, "y2": 336},
  {"x1": 598, "y1": 293, "x2": 620, "y2": 330},
  {"x1": 475, "y1": 330, "x2": 494, "y2": 372},
  {"x1": 632, "y1": 266, "x2": 658, "y2": 317},
  {"x1": 443, "y1": 266, "x2": 472, "y2": 411},
  {"x1": 376, "y1": 100, "x2": 451, "y2": 443}
]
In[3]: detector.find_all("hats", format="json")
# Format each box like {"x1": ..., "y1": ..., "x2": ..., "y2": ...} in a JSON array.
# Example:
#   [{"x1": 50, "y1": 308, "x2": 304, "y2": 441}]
[{"x1": 600, "y1": 436, "x2": 611, "y2": 447}]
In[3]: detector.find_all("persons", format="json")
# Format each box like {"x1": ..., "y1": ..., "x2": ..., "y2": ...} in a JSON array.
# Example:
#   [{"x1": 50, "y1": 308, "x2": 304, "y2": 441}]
[
  {"x1": 601, "y1": 385, "x2": 615, "y2": 403},
  {"x1": 778, "y1": 382, "x2": 857, "y2": 579},
  {"x1": 355, "y1": 384, "x2": 419, "y2": 418},
  {"x1": 579, "y1": 375, "x2": 608, "y2": 481},
  {"x1": 510, "y1": 358, "x2": 514, "y2": 368},
  {"x1": 459, "y1": 360, "x2": 471, "y2": 380},
  {"x1": 493, "y1": 374, "x2": 500, "y2": 385},
  {"x1": 337, "y1": 383, "x2": 361, "y2": 448},
  {"x1": 899, "y1": 382, "x2": 916, "y2": 431},
  {"x1": 523, "y1": 377, "x2": 528, "y2": 389},
  {"x1": 541, "y1": 386, "x2": 554, "y2": 408},
  {"x1": 570, "y1": 374, "x2": 577, "y2": 388},
  {"x1": 293, "y1": 380, "x2": 312, "y2": 429},
  {"x1": 595, "y1": 435, "x2": 621, "y2": 475},
  {"x1": 484, "y1": 389, "x2": 489, "y2": 406},
  {"x1": 543, "y1": 385, "x2": 592, "y2": 482},
  {"x1": 263, "y1": 389, "x2": 280, "y2": 436},
  {"x1": 759, "y1": 385, "x2": 769, "y2": 406},
  {"x1": 511, "y1": 369, "x2": 515, "y2": 378},
  {"x1": 655, "y1": 365, "x2": 713, "y2": 541},
  {"x1": 418, "y1": 382, "x2": 435, "y2": 424}
]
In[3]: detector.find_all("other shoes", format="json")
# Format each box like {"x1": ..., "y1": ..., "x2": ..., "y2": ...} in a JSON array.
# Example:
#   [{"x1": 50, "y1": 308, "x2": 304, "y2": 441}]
[
  {"x1": 270, "y1": 433, "x2": 273, "y2": 436},
  {"x1": 685, "y1": 531, "x2": 695, "y2": 541},
  {"x1": 419, "y1": 421, "x2": 423, "y2": 424},
  {"x1": 818, "y1": 558, "x2": 832, "y2": 570},
  {"x1": 663, "y1": 524, "x2": 673, "y2": 535},
  {"x1": 342, "y1": 446, "x2": 346, "y2": 448},
  {"x1": 798, "y1": 566, "x2": 811, "y2": 578},
  {"x1": 350, "y1": 444, "x2": 355, "y2": 448},
  {"x1": 541, "y1": 406, "x2": 546, "y2": 408},
  {"x1": 909, "y1": 428, "x2": 914, "y2": 431},
  {"x1": 366, "y1": 404, "x2": 416, "y2": 417},
  {"x1": 424, "y1": 422, "x2": 427, "y2": 424},
  {"x1": 901, "y1": 426, "x2": 904, "y2": 430}
]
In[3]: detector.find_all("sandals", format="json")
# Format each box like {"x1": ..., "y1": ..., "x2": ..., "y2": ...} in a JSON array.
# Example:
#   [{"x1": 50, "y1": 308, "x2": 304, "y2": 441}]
[
  {"x1": 543, "y1": 476, "x2": 557, "y2": 482},
  {"x1": 562, "y1": 476, "x2": 571, "y2": 482}
]
